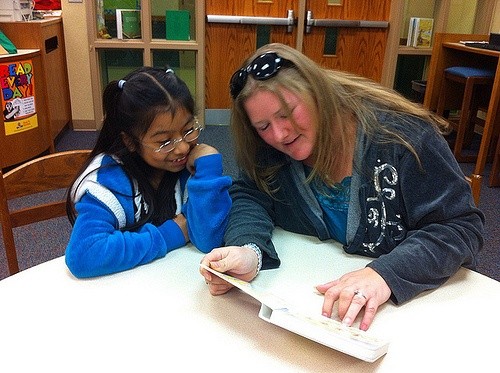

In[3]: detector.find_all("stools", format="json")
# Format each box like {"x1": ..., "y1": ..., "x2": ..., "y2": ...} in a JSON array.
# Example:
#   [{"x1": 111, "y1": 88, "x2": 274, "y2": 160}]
[{"x1": 437, "y1": 66, "x2": 498, "y2": 158}]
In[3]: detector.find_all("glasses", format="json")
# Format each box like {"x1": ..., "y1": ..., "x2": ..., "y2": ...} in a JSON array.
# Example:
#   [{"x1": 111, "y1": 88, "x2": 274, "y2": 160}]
[
  {"x1": 128, "y1": 115, "x2": 204, "y2": 154},
  {"x1": 228, "y1": 52, "x2": 297, "y2": 101}
]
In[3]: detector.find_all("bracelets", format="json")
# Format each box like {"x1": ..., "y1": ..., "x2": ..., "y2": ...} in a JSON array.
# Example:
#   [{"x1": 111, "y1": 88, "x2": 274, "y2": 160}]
[{"x1": 242, "y1": 242, "x2": 263, "y2": 276}]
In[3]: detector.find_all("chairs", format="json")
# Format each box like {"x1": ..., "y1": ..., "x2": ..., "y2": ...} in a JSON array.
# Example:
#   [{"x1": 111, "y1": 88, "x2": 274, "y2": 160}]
[{"x1": 0, "y1": 149, "x2": 94, "y2": 276}]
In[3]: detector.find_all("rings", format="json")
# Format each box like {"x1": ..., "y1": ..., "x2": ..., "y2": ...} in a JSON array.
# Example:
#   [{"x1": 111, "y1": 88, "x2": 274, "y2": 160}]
[{"x1": 354, "y1": 292, "x2": 367, "y2": 300}]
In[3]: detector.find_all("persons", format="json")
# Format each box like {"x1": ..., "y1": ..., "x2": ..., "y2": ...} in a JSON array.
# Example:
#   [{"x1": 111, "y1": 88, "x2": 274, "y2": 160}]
[
  {"x1": 203, "y1": 44, "x2": 485, "y2": 331},
  {"x1": 64, "y1": 65, "x2": 233, "y2": 279}
]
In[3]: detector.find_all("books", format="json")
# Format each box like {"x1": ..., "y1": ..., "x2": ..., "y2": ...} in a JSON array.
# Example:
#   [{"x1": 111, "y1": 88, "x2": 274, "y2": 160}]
[
  {"x1": 116, "y1": 8, "x2": 142, "y2": 40},
  {"x1": 203, "y1": 262, "x2": 390, "y2": 364},
  {"x1": 406, "y1": 16, "x2": 438, "y2": 48},
  {"x1": 444, "y1": 104, "x2": 461, "y2": 121}
]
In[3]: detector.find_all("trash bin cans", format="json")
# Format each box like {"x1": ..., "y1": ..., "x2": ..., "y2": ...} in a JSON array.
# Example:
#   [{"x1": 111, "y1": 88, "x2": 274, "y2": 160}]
[{"x1": 412, "y1": 80, "x2": 426, "y2": 102}]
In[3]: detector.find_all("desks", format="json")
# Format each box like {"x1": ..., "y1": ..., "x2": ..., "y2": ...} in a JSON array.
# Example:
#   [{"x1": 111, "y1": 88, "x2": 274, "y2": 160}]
[
  {"x1": 422, "y1": 32, "x2": 500, "y2": 176},
  {"x1": 0, "y1": 227, "x2": 500, "y2": 373}
]
